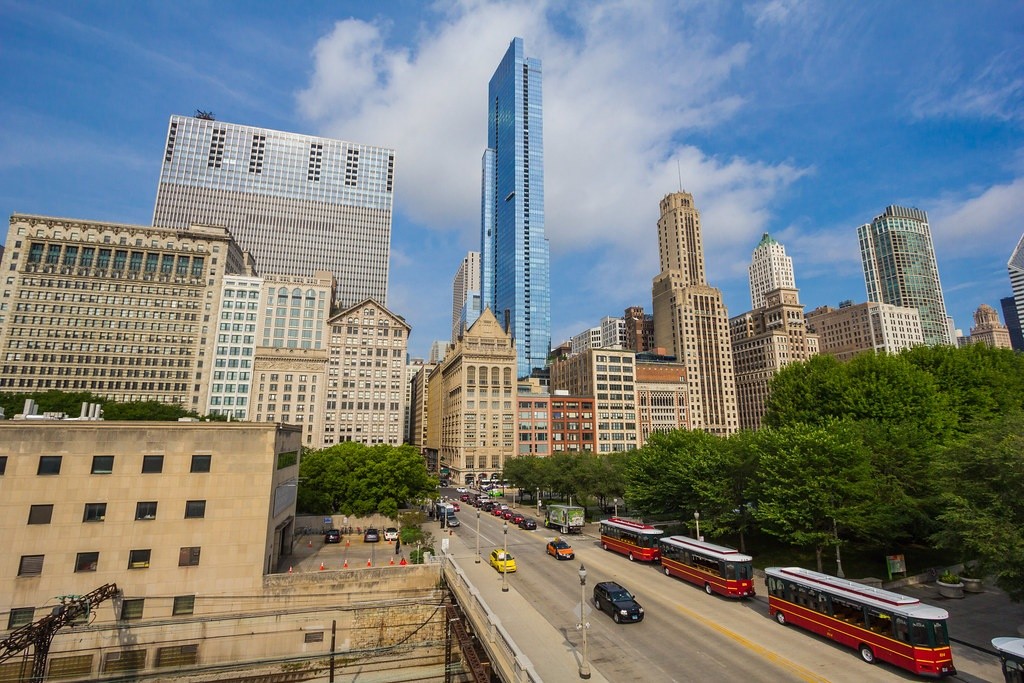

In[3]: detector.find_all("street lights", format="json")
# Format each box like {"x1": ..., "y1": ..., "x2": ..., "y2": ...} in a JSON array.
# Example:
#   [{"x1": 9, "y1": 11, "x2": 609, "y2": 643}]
[
  {"x1": 576, "y1": 563, "x2": 591, "y2": 679},
  {"x1": 614, "y1": 498, "x2": 617, "y2": 516},
  {"x1": 536, "y1": 486, "x2": 540, "y2": 517},
  {"x1": 475, "y1": 508, "x2": 481, "y2": 563},
  {"x1": 502, "y1": 521, "x2": 509, "y2": 592},
  {"x1": 694, "y1": 510, "x2": 700, "y2": 540}
]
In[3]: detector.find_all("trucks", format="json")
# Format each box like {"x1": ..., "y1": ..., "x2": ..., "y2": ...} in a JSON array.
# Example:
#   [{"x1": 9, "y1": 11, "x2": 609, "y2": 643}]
[{"x1": 543, "y1": 505, "x2": 585, "y2": 535}]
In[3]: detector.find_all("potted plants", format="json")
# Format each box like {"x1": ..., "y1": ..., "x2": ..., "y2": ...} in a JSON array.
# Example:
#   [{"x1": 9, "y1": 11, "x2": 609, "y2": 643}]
[
  {"x1": 957, "y1": 564, "x2": 984, "y2": 594},
  {"x1": 935, "y1": 568, "x2": 965, "y2": 600}
]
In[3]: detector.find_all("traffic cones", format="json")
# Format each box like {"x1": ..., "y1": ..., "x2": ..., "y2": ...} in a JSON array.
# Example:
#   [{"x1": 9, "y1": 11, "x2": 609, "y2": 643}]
[
  {"x1": 289, "y1": 566, "x2": 292, "y2": 573},
  {"x1": 388, "y1": 538, "x2": 393, "y2": 544},
  {"x1": 308, "y1": 539, "x2": 312, "y2": 547},
  {"x1": 346, "y1": 540, "x2": 350, "y2": 547},
  {"x1": 319, "y1": 555, "x2": 396, "y2": 570}
]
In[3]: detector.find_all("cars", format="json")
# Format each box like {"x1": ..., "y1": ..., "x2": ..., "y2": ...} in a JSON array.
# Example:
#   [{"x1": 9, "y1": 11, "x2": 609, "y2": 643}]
[
  {"x1": 325, "y1": 529, "x2": 342, "y2": 543},
  {"x1": 429, "y1": 495, "x2": 460, "y2": 528},
  {"x1": 546, "y1": 536, "x2": 575, "y2": 560},
  {"x1": 456, "y1": 484, "x2": 537, "y2": 530},
  {"x1": 384, "y1": 527, "x2": 399, "y2": 541},
  {"x1": 363, "y1": 528, "x2": 379, "y2": 542}
]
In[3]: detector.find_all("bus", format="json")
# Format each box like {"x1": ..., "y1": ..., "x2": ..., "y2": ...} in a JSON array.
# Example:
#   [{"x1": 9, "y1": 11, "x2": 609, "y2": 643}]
[
  {"x1": 990, "y1": 636, "x2": 1024, "y2": 683},
  {"x1": 764, "y1": 566, "x2": 957, "y2": 679},
  {"x1": 659, "y1": 535, "x2": 756, "y2": 599},
  {"x1": 598, "y1": 517, "x2": 664, "y2": 563},
  {"x1": 480, "y1": 479, "x2": 515, "y2": 491}
]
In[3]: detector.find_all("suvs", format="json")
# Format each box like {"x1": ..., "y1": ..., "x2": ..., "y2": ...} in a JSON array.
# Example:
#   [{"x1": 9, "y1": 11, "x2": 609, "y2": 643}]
[
  {"x1": 489, "y1": 548, "x2": 516, "y2": 574},
  {"x1": 593, "y1": 581, "x2": 645, "y2": 624}
]
